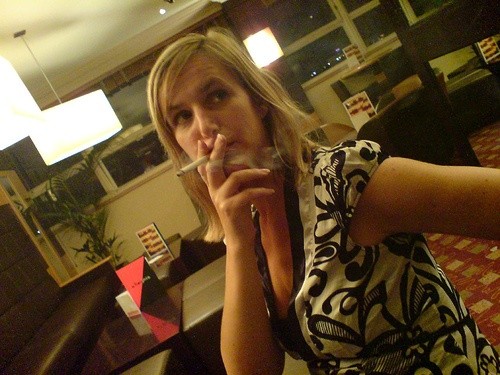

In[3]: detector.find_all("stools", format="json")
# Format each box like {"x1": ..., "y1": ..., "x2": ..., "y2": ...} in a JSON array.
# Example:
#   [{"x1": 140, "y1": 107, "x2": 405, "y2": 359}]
[{"x1": 182, "y1": 253, "x2": 227, "y2": 375}]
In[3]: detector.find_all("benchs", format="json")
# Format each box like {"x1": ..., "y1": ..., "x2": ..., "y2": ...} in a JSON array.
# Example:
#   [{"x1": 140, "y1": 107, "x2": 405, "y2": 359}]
[{"x1": 0, "y1": 186, "x2": 123, "y2": 375}]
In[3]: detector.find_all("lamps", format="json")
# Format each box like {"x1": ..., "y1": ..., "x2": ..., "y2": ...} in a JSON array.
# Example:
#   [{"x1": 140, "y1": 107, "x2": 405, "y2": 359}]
[
  {"x1": 13, "y1": 30, "x2": 123, "y2": 167},
  {"x1": 242, "y1": 26, "x2": 285, "y2": 71},
  {"x1": 0, "y1": 56, "x2": 42, "y2": 150}
]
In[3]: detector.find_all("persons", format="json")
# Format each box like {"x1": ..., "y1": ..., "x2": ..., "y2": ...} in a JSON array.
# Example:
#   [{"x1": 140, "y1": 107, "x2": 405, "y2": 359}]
[{"x1": 144, "y1": 26, "x2": 500, "y2": 375}]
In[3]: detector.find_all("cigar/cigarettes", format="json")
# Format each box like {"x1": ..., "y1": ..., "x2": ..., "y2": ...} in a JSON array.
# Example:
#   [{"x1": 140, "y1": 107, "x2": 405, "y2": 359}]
[{"x1": 176, "y1": 154, "x2": 210, "y2": 178}]
